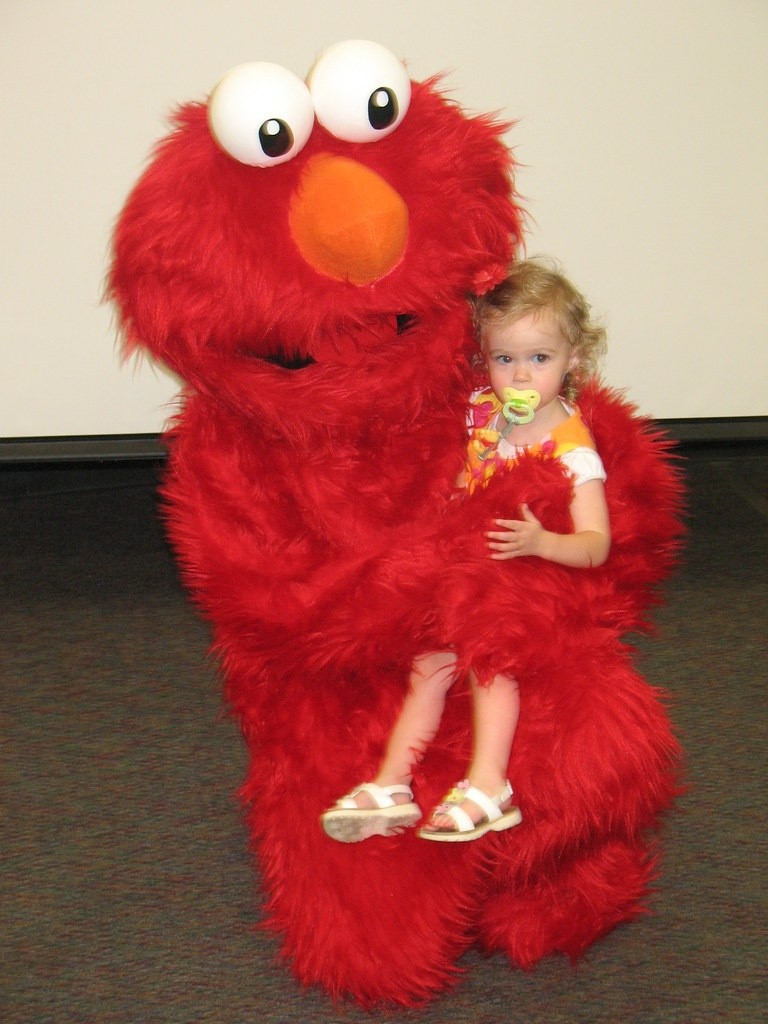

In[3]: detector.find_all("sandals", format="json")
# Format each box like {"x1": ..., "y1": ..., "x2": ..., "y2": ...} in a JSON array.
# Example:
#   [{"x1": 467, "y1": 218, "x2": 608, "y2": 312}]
[
  {"x1": 418, "y1": 780, "x2": 523, "y2": 843},
  {"x1": 322, "y1": 783, "x2": 423, "y2": 842}
]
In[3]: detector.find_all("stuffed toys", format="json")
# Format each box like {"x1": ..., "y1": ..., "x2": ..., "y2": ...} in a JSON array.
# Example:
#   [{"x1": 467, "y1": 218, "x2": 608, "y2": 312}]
[{"x1": 110, "y1": 36, "x2": 690, "y2": 1011}]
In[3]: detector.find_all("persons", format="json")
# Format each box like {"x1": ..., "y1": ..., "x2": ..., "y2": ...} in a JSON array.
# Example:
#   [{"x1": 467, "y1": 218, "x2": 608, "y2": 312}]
[{"x1": 321, "y1": 264, "x2": 612, "y2": 841}]
